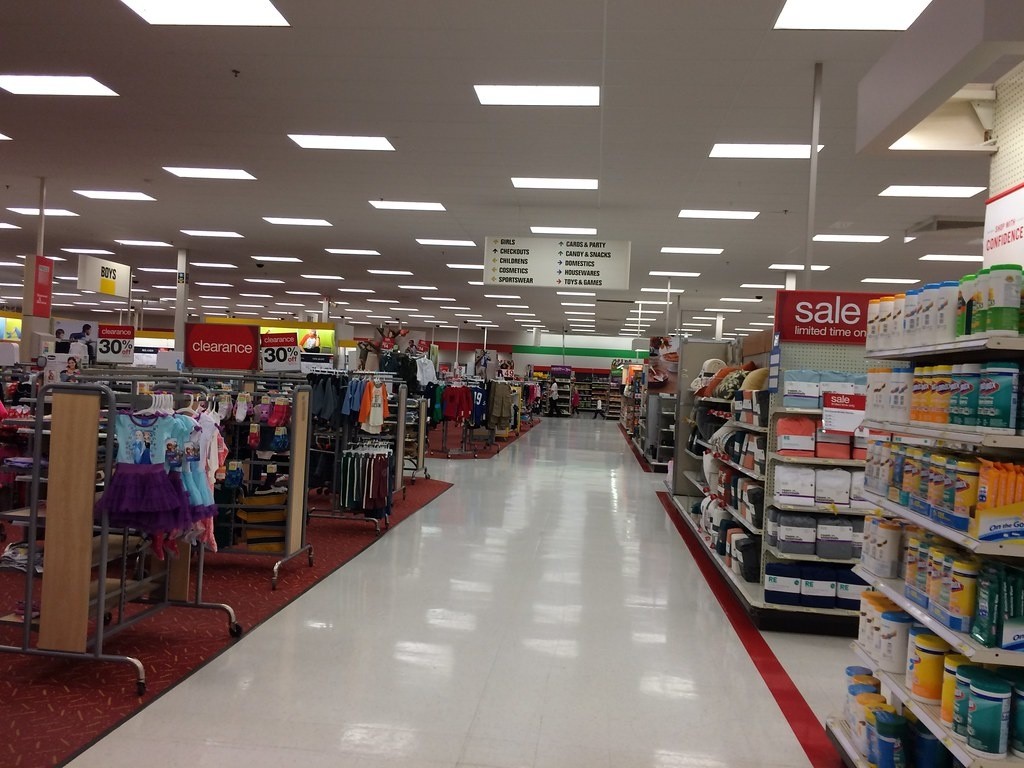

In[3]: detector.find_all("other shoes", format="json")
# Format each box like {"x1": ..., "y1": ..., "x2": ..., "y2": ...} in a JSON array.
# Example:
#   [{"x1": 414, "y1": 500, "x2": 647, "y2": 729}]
[
  {"x1": 592, "y1": 417, "x2": 596, "y2": 419},
  {"x1": 603, "y1": 416, "x2": 606, "y2": 420}
]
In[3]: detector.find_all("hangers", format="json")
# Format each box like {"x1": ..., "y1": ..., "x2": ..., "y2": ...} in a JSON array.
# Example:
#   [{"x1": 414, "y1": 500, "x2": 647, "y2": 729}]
[
  {"x1": 523, "y1": 381, "x2": 540, "y2": 387},
  {"x1": 435, "y1": 379, "x2": 483, "y2": 389},
  {"x1": 492, "y1": 376, "x2": 512, "y2": 385},
  {"x1": 132, "y1": 394, "x2": 217, "y2": 417},
  {"x1": 343, "y1": 439, "x2": 393, "y2": 458}
]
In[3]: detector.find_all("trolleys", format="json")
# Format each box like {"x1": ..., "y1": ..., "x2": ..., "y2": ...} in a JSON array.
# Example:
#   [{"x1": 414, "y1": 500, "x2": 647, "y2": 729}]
[{"x1": 540, "y1": 392, "x2": 549, "y2": 415}]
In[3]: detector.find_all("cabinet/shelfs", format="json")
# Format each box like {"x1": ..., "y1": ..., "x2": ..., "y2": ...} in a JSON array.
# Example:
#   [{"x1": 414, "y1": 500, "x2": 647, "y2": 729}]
[
  {"x1": 529, "y1": 290, "x2": 899, "y2": 637},
  {"x1": 0, "y1": 382, "x2": 243, "y2": 695},
  {"x1": 312, "y1": 369, "x2": 382, "y2": 384},
  {"x1": 313, "y1": 399, "x2": 431, "y2": 485},
  {"x1": 824, "y1": 338, "x2": 1024, "y2": 768}
]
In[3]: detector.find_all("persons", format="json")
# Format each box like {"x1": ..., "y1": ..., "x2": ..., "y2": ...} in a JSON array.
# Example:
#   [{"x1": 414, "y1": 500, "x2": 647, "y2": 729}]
[
  {"x1": 81, "y1": 324, "x2": 93, "y2": 341},
  {"x1": 498, "y1": 360, "x2": 514, "y2": 378},
  {"x1": 476, "y1": 350, "x2": 491, "y2": 378},
  {"x1": 68, "y1": 357, "x2": 78, "y2": 373},
  {"x1": 592, "y1": 396, "x2": 605, "y2": 420},
  {"x1": 547, "y1": 377, "x2": 562, "y2": 418},
  {"x1": 56, "y1": 329, "x2": 64, "y2": 342},
  {"x1": 569, "y1": 386, "x2": 580, "y2": 419},
  {"x1": 299, "y1": 331, "x2": 321, "y2": 353},
  {"x1": 405, "y1": 340, "x2": 417, "y2": 354}
]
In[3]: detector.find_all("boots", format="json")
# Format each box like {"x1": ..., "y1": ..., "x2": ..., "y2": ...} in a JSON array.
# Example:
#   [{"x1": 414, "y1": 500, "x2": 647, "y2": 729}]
[
  {"x1": 576, "y1": 414, "x2": 580, "y2": 419},
  {"x1": 569, "y1": 414, "x2": 574, "y2": 418}
]
